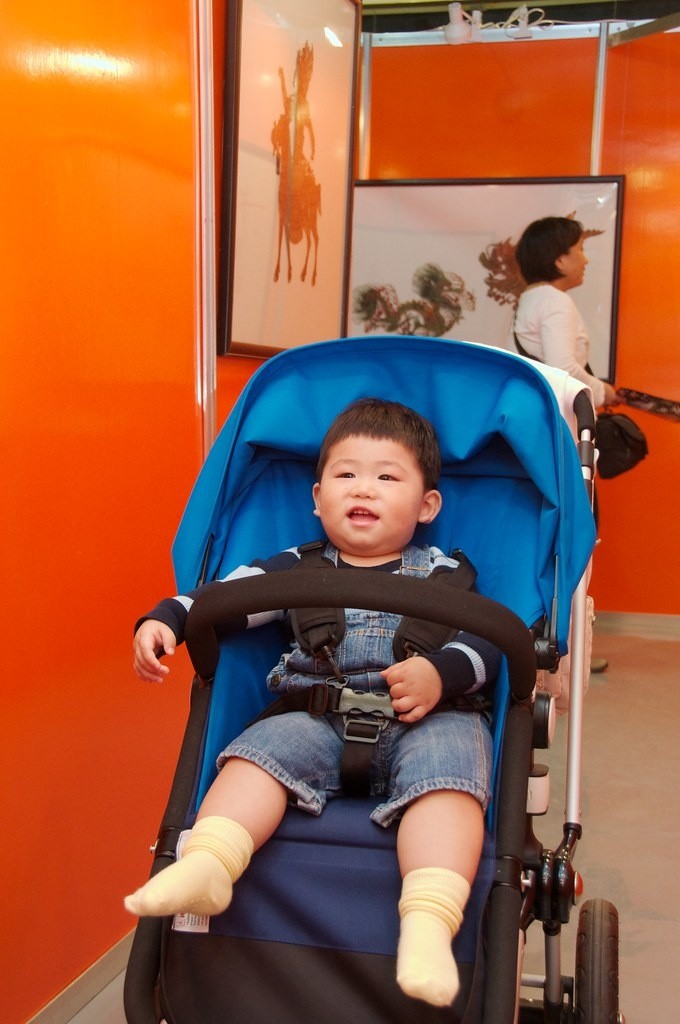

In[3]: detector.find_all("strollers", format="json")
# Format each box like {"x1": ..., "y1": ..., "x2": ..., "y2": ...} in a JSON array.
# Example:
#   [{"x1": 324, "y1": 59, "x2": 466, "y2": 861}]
[{"x1": 121, "y1": 332, "x2": 620, "y2": 1024}]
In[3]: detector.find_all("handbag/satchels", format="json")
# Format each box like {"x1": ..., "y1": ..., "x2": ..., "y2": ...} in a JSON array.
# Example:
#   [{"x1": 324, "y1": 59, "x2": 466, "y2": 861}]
[{"x1": 594, "y1": 412, "x2": 649, "y2": 479}]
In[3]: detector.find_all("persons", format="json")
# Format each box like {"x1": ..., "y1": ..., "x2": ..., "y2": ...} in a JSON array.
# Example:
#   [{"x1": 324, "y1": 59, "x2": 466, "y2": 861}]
[
  {"x1": 509, "y1": 216, "x2": 615, "y2": 673},
  {"x1": 119, "y1": 398, "x2": 500, "y2": 1009}
]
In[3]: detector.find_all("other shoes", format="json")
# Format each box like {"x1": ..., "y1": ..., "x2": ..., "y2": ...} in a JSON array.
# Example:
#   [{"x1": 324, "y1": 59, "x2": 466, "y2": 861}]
[{"x1": 590, "y1": 658, "x2": 608, "y2": 674}]
